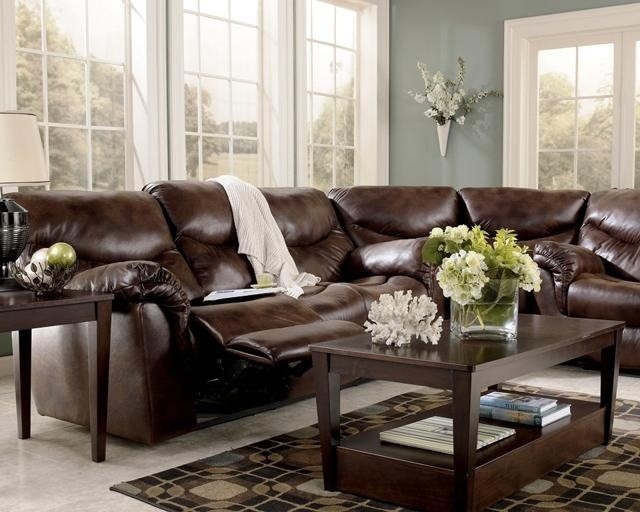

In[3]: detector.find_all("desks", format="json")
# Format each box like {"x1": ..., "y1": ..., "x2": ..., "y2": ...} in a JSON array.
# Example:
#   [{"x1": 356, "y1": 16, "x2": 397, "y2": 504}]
[{"x1": 0, "y1": 287, "x2": 115, "y2": 462}]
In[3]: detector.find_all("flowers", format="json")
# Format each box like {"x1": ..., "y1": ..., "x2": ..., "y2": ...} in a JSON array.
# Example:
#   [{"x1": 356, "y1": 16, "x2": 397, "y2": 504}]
[{"x1": 405, "y1": 55, "x2": 503, "y2": 125}]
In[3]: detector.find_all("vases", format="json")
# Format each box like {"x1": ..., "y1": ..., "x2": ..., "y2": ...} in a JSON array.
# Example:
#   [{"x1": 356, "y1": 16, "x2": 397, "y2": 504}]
[{"x1": 435, "y1": 120, "x2": 451, "y2": 159}]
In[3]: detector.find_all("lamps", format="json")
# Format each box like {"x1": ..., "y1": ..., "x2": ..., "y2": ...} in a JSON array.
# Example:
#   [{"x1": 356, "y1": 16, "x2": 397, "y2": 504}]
[{"x1": 0, "y1": 111, "x2": 51, "y2": 292}]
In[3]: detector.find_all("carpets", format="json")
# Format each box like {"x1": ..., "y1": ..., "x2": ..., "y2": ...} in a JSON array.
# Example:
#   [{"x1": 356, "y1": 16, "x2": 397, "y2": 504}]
[{"x1": 108, "y1": 381, "x2": 640, "y2": 511}]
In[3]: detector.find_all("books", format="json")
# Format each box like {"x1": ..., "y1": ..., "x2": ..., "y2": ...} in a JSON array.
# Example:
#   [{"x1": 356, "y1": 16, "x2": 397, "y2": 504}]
[
  {"x1": 475, "y1": 405, "x2": 569, "y2": 428},
  {"x1": 378, "y1": 416, "x2": 515, "y2": 454},
  {"x1": 480, "y1": 391, "x2": 556, "y2": 413}
]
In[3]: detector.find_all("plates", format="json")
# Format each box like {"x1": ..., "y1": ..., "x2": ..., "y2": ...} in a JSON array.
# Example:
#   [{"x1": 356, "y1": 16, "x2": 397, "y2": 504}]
[{"x1": 250, "y1": 283, "x2": 277, "y2": 289}]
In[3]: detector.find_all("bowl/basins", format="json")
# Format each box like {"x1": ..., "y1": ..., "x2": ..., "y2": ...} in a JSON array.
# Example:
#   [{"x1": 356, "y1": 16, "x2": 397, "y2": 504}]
[{"x1": 6, "y1": 258, "x2": 80, "y2": 298}]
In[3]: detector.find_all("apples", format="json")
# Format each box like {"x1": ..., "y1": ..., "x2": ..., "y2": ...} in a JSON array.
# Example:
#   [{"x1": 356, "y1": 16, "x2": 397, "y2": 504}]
[{"x1": 46, "y1": 242, "x2": 77, "y2": 267}]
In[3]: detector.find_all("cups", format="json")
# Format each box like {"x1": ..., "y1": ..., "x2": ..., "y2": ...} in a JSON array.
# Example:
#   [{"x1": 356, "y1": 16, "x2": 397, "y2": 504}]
[{"x1": 256, "y1": 273, "x2": 272, "y2": 284}]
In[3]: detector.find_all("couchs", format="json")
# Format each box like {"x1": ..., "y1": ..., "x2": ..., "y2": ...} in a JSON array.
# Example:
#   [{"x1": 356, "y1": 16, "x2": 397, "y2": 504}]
[{"x1": 4, "y1": 182, "x2": 640, "y2": 447}]
